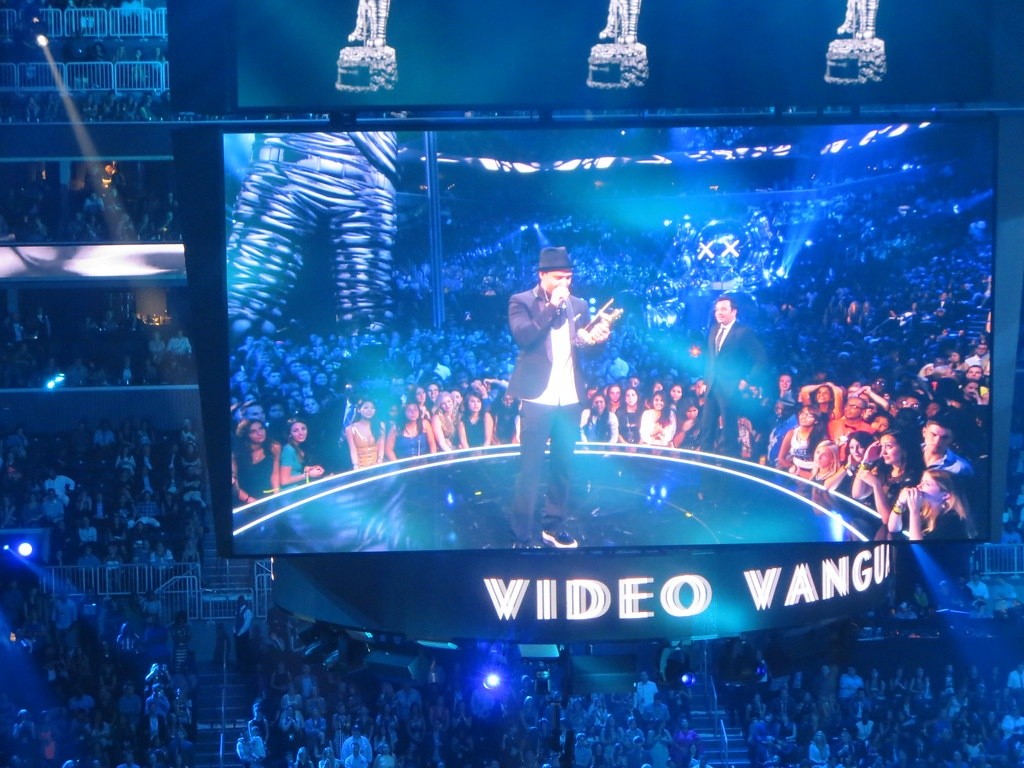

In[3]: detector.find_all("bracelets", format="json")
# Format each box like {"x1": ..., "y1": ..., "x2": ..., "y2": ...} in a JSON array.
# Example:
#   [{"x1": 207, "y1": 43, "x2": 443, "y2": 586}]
[
  {"x1": 860, "y1": 462, "x2": 874, "y2": 471},
  {"x1": 893, "y1": 503, "x2": 907, "y2": 516}
]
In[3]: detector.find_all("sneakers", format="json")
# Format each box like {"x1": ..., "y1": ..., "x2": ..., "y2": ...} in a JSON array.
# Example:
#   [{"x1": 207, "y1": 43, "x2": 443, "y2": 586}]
[
  {"x1": 542, "y1": 529, "x2": 578, "y2": 548},
  {"x1": 511, "y1": 531, "x2": 543, "y2": 548}
]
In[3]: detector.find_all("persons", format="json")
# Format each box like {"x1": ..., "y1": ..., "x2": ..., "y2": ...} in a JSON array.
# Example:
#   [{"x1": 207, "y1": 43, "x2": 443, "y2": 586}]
[
  {"x1": 507, "y1": 247, "x2": 610, "y2": 549},
  {"x1": 1001, "y1": 414, "x2": 1024, "y2": 546},
  {"x1": 1, "y1": 0, "x2": 210, "y2": 768},
  {"x1": 229, "y1": 170, "x2": 991, "y2": 540},
  {"x1": 232, "y1": 570, "x2": 1024, "y2": 768}
]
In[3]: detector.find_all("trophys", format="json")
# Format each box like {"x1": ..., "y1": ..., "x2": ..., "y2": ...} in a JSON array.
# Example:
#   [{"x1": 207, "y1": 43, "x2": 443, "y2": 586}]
[{"x1": 574, "y1": 297, "x2": 624, "y2": 349}]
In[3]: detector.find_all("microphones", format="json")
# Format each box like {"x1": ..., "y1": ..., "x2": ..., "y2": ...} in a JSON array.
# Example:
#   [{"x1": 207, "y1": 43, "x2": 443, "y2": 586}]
[{"x1": 560, "y1": 302, "x2": 567, "y2": 315}]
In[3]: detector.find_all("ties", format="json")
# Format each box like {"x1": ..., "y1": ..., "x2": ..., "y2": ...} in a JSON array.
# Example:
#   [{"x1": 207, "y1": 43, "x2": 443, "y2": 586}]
[{"x1": 714, "y1": 328, "x2": 726, "y2": 357}]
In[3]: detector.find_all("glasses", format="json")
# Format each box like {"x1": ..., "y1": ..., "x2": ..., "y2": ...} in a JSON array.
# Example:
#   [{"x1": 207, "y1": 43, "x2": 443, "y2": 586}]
[
  {"x1": 874, "y1": 381, "x2": 886, "y2": 387},
  {"x1": 799, "y1": 412, "x2": 814, "y2": 418},
  {"x1": 844, "y1": 404, "x2": 862, "y2": 410},
  {"x1": 901, "y1": 401, "x2": 918, "y2": 408}
]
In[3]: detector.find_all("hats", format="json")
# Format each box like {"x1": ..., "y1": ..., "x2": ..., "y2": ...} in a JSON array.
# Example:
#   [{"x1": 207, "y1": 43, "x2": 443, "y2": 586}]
[
  {"x1": 352, "y1": 724, "x2": 361, "y2": 732},
  {"x1": 535, "y1": 247, "x2": 576, "y2": 270}
]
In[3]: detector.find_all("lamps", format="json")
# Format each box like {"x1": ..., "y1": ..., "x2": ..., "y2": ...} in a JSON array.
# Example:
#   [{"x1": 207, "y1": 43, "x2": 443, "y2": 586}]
[
  {"x1": 320, "y1": 646, "x2": 346, "y2": 673},
  {"x1": 301, "y1": 639, "x2": 328, "y2": 664},
  {"x1": 334, "y1": 0, "x2": 399, "y2": 94},
  {"x1": 663, "y1": 649, "x2": 698, "y2": 692},
  {"x1": 731, "y1": 648, "x2": 766, "y2": 686},
  {"x1": 587, "y1": 0, "x2": 649, "y2": 90},
  {"x1": 480, "y1": 654, "x2": 508, "y2": 692},
  {"x1": 823, "y1": 0, "x2": 888, "y2": 87}
]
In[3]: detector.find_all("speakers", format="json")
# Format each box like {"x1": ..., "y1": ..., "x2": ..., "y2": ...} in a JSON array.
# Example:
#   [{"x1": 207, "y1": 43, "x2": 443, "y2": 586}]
[
  {"x1": 570, "y1": 655, "x2": 638, "y2": 694},
  {"x1": 362, "y1": 650, "x2": 424, "y2": 684}
]
